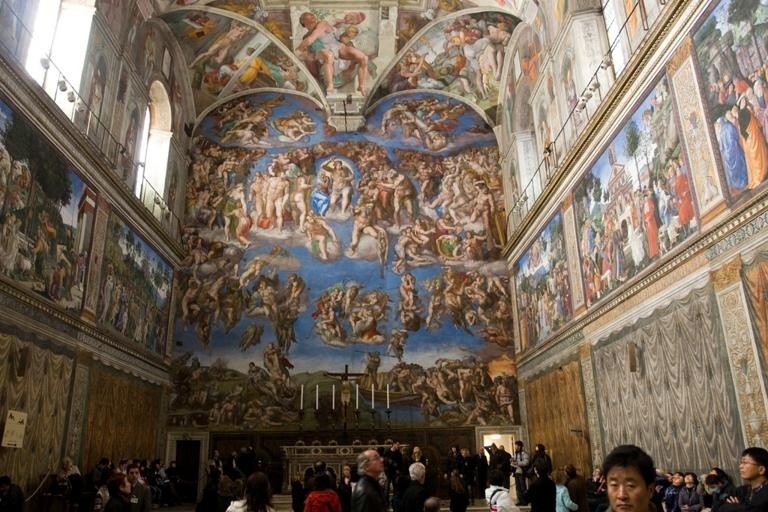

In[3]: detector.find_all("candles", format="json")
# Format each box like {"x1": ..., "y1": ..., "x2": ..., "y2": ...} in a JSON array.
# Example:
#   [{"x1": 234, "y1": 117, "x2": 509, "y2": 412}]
[
  {"x1": 315, "y1": 383, "x2": 319, "y2": 410},
  {"x1": 355, "y1": 383, "x2": 359, "y2": 410},
  {"x1": 386, "y1": 383, "x2": 390, "y2": 409},
  {"x1": 300, "y1": 384, "x2": 305, "y2": 410},
  {"x1": 371, "y1": 383, "x2": 375, "y2": 409},
  {"x1": 332, "y1": 383, "x2": 335, "y2": 410}
]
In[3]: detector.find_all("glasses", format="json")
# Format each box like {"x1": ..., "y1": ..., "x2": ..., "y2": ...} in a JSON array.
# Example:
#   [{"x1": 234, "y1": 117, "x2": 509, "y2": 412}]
[{"x1": 738, "y1": 459, "x2": 762, "y2": 467}]
